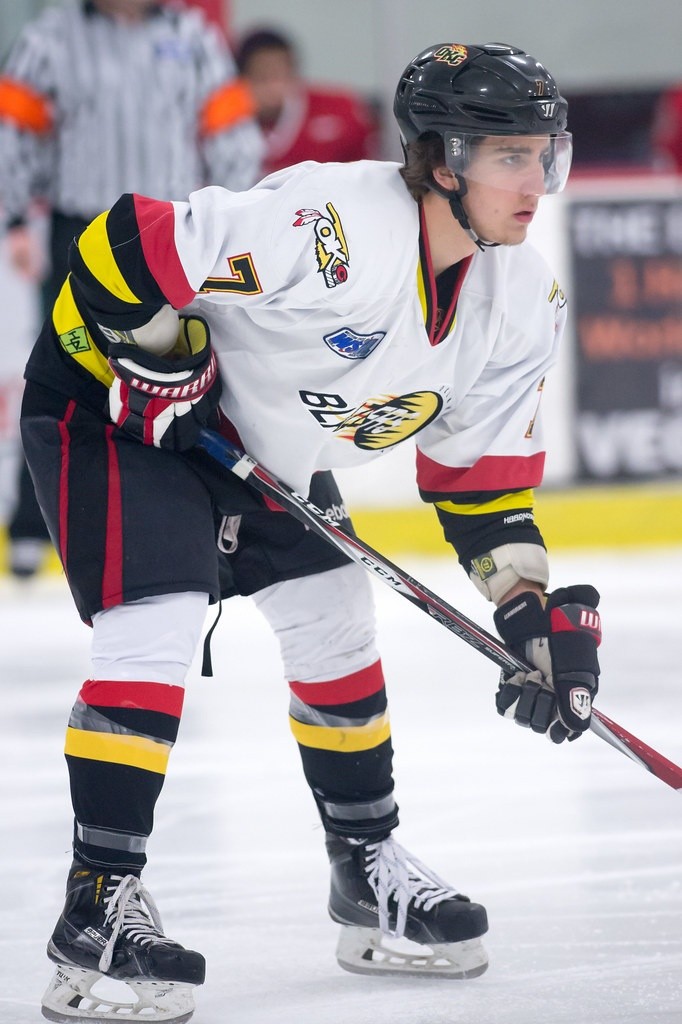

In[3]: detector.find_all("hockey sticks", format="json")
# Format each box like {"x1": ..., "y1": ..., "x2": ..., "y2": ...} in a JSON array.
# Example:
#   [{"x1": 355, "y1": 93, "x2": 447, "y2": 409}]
[{"x1": 196, "y1": 426, "x2": 682, "y2": 794}]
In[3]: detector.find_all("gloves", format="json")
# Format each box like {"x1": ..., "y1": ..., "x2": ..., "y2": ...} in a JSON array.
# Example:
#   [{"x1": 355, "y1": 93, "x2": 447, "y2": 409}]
[
  {"x1": 495, "y1": 584, "x2": 602, "y2": 744},
  {"x1": 109, "y1": 314, "x2": 223, "y2": 455}
]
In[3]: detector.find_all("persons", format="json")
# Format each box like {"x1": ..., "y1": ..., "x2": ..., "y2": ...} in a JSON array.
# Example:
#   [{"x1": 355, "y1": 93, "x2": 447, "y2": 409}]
[
  {"x1": 0, "y1": 0, "x2": 382, "y2": 580},
  {"x1": 14, "y1": 44, "x2": 606, "y2": 986}
]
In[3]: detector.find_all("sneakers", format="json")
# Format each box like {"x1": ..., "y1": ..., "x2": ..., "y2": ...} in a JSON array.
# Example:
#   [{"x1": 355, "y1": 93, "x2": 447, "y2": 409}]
[
  {"x1": 10, "y1": 539, "x2": 40, "y2": 577},
  {"x1": 41, "y1": 859, "x2": 206, "y2": 1024},
  {"x1": 324, "y1": 835, "x2": 491, "y2": 979}
]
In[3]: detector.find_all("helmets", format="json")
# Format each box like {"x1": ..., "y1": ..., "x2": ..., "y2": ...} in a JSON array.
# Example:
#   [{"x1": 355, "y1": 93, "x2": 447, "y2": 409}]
[{"x1": 393, "y1": 42, "x2": 570, "y2": 200}]
